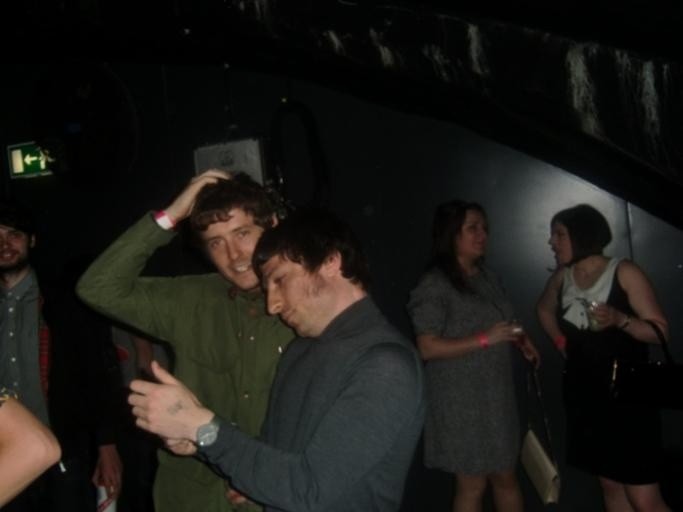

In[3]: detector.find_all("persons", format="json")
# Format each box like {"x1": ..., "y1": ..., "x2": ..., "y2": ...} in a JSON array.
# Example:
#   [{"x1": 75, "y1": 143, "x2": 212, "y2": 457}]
[
  {"x1": 532, "y1": 203, "x2": 673, "y2": 511},
  {"x1": 126, "y1": 205, "x2": 427, "y2": 512},
  {"x1": 0, "y1": 215, "x2": 175, "y2": 512},
  {"x1": 73, "y1": 166, "x2": 296, "y2": 512},
  {"x1": 404, "y1": 197, "x2": 543, "y2": 512}
]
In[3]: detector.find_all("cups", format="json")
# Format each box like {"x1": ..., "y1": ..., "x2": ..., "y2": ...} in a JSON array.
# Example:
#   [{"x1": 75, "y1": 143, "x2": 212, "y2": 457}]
[
  {"x1": 97, "y1": 481, "x2": 117, "y2": 512},
  {"x1": 584, "y1": 300, "x2": 604, "y2": 332}
]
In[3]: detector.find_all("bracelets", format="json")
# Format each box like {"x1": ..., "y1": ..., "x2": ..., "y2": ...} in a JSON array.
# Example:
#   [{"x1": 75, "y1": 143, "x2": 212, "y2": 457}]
[{"x1": 477, "y1": 332, "x2": 488, "y2": 350}]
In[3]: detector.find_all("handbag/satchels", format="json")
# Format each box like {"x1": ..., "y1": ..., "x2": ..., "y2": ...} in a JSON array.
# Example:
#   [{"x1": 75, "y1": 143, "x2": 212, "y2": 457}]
[
  {"x1": 518, "y1": 360, "x2": 562, "y2": 504},
  {"x1": 612, "y1": 318, "x2": 683, "y2": 412}
]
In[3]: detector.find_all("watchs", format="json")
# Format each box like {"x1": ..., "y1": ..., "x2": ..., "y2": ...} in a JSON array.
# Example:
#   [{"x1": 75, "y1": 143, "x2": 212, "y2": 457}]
[
  {"x1": 192, "y1": 415, "x2": 224, "y2": 451},
  {"x1": 618, "y1": 312, "x2": 631, "y2": 330}
]
